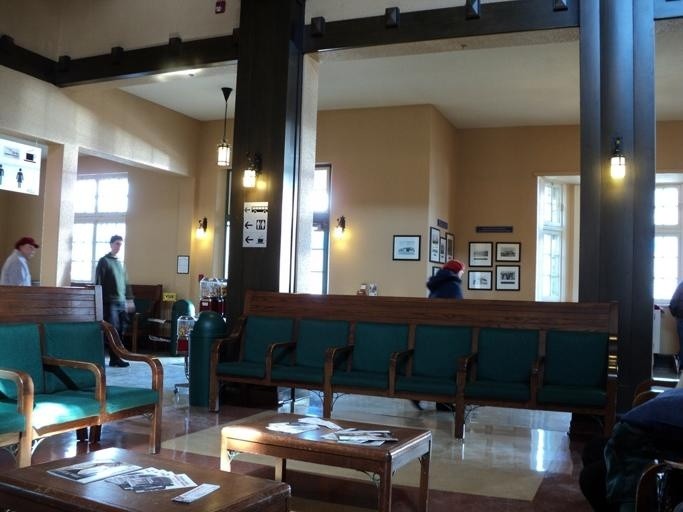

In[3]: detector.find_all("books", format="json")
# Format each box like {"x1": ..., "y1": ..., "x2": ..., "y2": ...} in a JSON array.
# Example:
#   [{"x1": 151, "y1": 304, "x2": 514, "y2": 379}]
[
  {"x1": 265, "y1": 416, "x2": 399, "y2": 448},
  {"x1": 46, "y1": 458, "x2": 197, "y2": 493}
]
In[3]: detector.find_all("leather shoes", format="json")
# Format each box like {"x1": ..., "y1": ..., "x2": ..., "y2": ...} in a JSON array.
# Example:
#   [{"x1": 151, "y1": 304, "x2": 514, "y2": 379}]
[
  {"x1": 436, "y1": 403, "x2": 456, "y2": 413},
  {"x1": 109, "y1": 357, "x2": 129, "y2": 368}
]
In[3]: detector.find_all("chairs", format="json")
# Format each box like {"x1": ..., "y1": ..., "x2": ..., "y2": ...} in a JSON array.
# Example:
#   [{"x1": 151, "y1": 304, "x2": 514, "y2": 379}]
[
  {"x1": 1, "y1": 282, "x2": 164, "y2": 469},
  {"x1": 208, "y1": 291, "x2": 618, "y2": 437},
  {"x1": 623, "y1": 375, "x2": 683, "y2": 511}
]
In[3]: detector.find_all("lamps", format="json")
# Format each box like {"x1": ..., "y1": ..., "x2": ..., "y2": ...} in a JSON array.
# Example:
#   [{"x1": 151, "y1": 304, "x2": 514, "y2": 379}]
[
  {"x1": 195, "y1": 216, "x2": 208, "y2": 239},
  {"x1": 241, "y1": 150, "x2": 263, "y2": 188},
  {"x1": 333, "y1": 216, "x2": 345, "y2": 238},
  {"x1": 608, "y1": 136, "x2": 629, "y2": 182},
  {"x1": 215, "y1": 86, "x2": 233, "y2": 168}
]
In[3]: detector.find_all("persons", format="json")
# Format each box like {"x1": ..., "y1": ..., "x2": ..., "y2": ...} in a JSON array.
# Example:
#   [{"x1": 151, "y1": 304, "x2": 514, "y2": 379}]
[
  {"x1": 1, "y1": 235, "x2": 40, "y2": 286},
  {"x1": 409, "y1": 260, "x2": 468, "y2": 413},
  {"x1": 598, "y1": 279, "x2": 682, "y2": 511},
  {"x1": 94, "y1": 233, "x2": 135, "y2": 367}
]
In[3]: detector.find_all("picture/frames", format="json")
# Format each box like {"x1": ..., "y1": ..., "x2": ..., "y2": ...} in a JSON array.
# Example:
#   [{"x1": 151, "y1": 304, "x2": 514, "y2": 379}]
[
  {"x1": 429, "y1": 227, "x2": 440, "y2": 263},
  {"x1": 468, "y1": 242, "x2": 493, "y2": 267},
  {"x1": 468, "y1": 270, "x2": 492, "y2": 290},
  {"x1": 432, "y1": 266, "x2": 441, "y2": 276},
  {"x1": 176, "y1": 254, "x2": 190, "y2": 274},
  {"x1": 495, "y1": 264, "x2": 520, "y2": 291},
  {"x1": 495, "y1": 242, "x2": 521, "y2": 262},
  {"x1": 439, "y1": 237, "x2": 447, "y2": 263},
  {"x1": 445, "y1": 233, "x2": 455, "y2": 264},
  {"x1": 392, "y1": 235, "x2": 421, "y2": 261}
]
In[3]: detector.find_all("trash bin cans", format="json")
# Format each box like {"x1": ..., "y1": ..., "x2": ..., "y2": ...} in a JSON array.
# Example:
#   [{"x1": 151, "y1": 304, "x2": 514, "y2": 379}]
[
  {"x1": 169, "y1": 299, "x2": 195, "y2": 357},
  {"x1": 188, "y1": 310, "x2": 228, "y2": 408}
]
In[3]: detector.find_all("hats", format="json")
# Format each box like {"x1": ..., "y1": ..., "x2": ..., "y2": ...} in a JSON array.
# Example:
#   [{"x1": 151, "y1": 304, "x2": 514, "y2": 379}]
[
  {"x1": 442, "y1": 259, "x2": 465, "y2": 273},
  {"x1": 16, "y1": 237, "x2": 39, "y2": 251}
]
